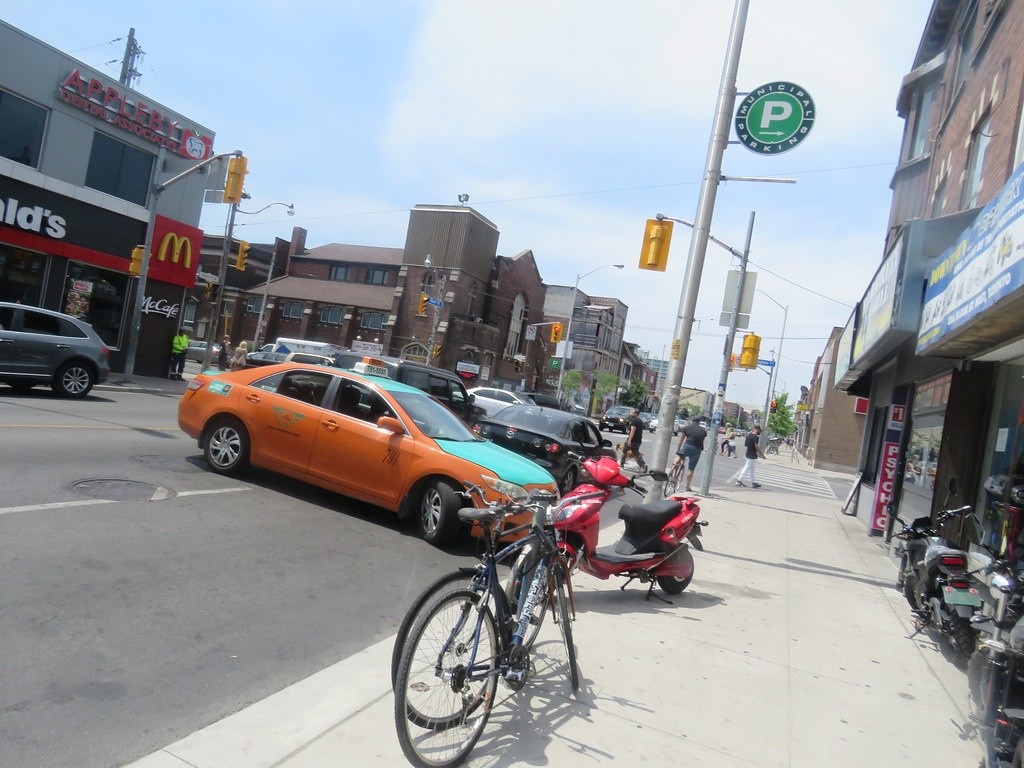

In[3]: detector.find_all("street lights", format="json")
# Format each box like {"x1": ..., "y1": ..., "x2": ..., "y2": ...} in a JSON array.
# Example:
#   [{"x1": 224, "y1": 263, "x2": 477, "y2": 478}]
[
  {"x1": 760, "y1": 349, "x2": 776, "y2": 443},
  {"x1": 423, "y1": 254, "x2": 447, "y2": 365},
  {"x1": 651, "y1": 338, "x2": 694, "y2": 414},
  {"x1": 554, "y1": 262, "x2": 626, "y2": 399},
  {"x1": 195, "y1": 196, "x2": 296, "y2": 377}
]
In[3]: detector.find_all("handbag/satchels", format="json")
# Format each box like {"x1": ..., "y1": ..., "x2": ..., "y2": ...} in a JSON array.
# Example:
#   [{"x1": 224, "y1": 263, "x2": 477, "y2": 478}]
[{"x1": 239, "y1": 354, "x2": 246, "y2": 367}]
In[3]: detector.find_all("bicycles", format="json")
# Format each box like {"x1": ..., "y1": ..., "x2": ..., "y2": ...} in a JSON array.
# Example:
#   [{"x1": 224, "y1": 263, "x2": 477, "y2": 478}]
[
  {"x1": 391, "y1": 479, "x2": 608, "y2": 768},
  {"x1": 662, "y1": 447, "x2": 689, "y2": 498}
]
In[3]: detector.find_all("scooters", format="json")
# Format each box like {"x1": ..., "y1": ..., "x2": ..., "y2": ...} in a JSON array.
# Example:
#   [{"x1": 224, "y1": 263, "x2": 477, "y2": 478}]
[{"x1": 537, "y1": 421, "x2": 709, "y2": 600}]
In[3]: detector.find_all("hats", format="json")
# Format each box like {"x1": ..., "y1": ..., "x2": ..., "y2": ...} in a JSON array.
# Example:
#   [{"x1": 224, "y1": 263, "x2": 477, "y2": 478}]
[{"x1": 693, "y1": 416, "x2": 699, "y2": 422}]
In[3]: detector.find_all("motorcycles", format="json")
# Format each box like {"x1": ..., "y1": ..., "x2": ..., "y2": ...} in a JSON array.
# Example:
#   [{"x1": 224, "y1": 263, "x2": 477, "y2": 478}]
[
  {"x1": 955, "y1": 510, "x2": 1024, "y2": 768},
  {"x1": 880, "y1": 476, "x2": 986, "y2": 670}
]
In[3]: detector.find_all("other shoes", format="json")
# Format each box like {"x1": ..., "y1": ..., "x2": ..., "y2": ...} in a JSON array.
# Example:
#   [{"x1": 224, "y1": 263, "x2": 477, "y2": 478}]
[
  {"x1": 752, "y1": 483, "x2": 761, "y2": 488},
  {"x1": 618, "y1": 465, "x2": 623, "y2": 469},
  {"x1": 685, "y1": 487, "x2": 691, "y2": 492},
  {"x1": 170, "y1": 376, "x2": 177, "y2": 381},
  {"x1": 643, "y1": 464, "x2": 647, "y2": 473},
  {"x1": 178, "y1": 376, "x2": 186, "y2": 381},
  {"x1": 737, "y1": 481, "x2": 746, "y2": 488}
]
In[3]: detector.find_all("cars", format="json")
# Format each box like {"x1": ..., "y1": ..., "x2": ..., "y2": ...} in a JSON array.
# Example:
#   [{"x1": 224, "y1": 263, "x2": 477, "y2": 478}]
[
  {"x1": 598, "y1": 404, "x2": 637, "y2": 433},
  {"x1": 184, "y1": 340, "x2": 223, "y2": 367},
  {"x1": 240, "y1": 333, "x2": 587, "y2": 449},
  {"x1": 0, "y1": 299, "x2": 112, "y2": 399},
  {"x1": 470, "y1": 404, "x2": 617, "y2": 499},
  {"x1": 638, "y1": 411, "x2": 753, "y2": 439},
  {"x1": 179, "y1": 354, "x2": 560, "y2": 551}
]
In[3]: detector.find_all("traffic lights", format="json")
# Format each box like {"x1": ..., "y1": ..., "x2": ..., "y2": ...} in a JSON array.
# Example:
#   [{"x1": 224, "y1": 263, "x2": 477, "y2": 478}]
[
  {"x1": 236, "y1": 237, "x2": 251, "y2": 271},
  {"x1": 431, "y1": 343, "x2": 443, "y2": 358},
  {"x1": 515, "y1": 361, "x2": 524, "y2": 373},
  {"x1": 770, "y1": 402, "x2": 778, "y2": 414},
  {"x1": 550, "y1": 323, "x2": 564, "y2": 344},
  {"x1": 417, "y1": 291, "x2": 430, "y2": 318},
  {"x1": 206, "y1": 280, "x2": 222, "y2": 302}
]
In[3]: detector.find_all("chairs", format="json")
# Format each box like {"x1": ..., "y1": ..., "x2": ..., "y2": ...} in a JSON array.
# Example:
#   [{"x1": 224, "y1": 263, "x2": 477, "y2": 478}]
[{"x1": 282, "y1": 380, "x2": 388, "y2": 421}]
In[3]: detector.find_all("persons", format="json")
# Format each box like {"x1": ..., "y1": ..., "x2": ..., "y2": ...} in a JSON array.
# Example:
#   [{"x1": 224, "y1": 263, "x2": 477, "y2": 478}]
[
  {"x1": 671, "y1": 417, "x2": 707, "y2": 491},
  {"x1": 230, "y1": 341, "x2": 248, "y2": 370},
  {"x1": 785, "y1": 436, "x2": 797, "y2": 450},
  {"x1": 720, "y1": 422, "x2": 738, "y2": 459},
  {"x1": 171, "y1": 328, "x2": 188, "y2": 381},
  {"x1": 930, "y1": 447, "x2": 936, "y2": 461},
  {"x1": 908, "y1": 455, "x2": 920, "y2": 479},
  {"x1": 736, "y1": 425, "x2": 766, "y2": 488},
  {"x1": 219, "y1": 335, "x2": 230, "y2": 371},
  {"x1": 619, "y1": 409, "x2": 648, "y2": 473}
]
